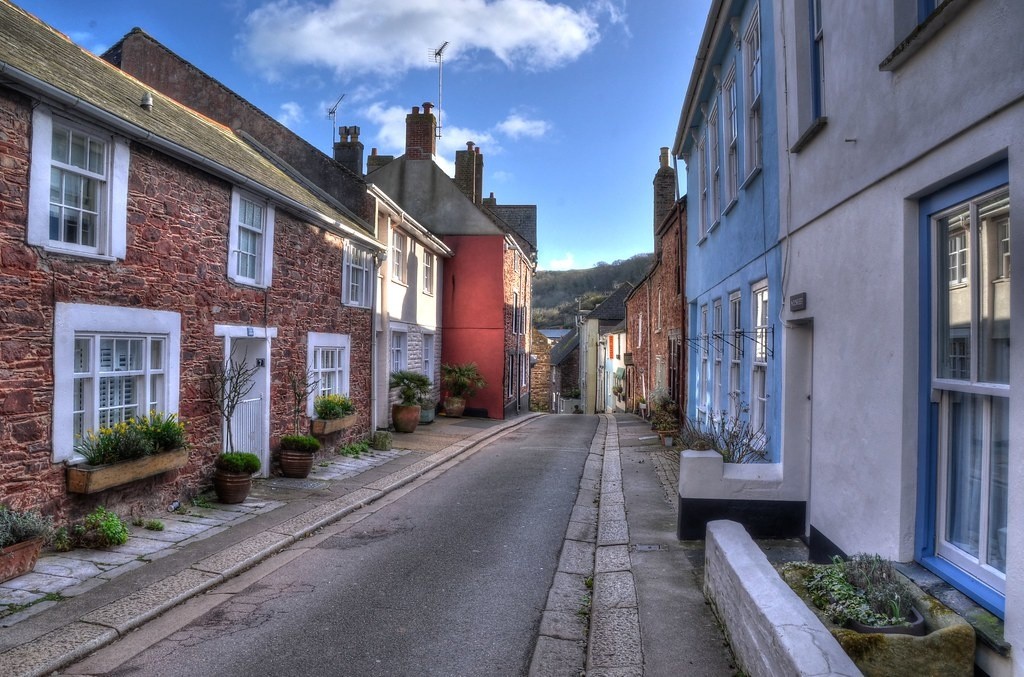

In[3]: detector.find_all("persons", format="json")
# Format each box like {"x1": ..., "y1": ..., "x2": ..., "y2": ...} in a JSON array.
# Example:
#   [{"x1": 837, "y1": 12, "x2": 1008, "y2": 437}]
[{"x1": 572, "y1": 405, "x2": 583, "y2": 414}]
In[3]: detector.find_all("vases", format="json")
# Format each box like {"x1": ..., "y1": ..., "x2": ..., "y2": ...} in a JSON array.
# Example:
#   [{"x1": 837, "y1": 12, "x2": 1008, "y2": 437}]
[
  {"x1": 66, "y1": 444, "x2": 189, "y2": 495},
  {"x1": 311, "y1": 413, "x2": 358, "y2": 435}
]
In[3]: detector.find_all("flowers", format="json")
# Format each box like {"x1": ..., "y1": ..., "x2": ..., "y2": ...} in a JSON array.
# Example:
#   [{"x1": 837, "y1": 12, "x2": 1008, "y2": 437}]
[
  {"x1": 74, "y1": 409, "x2": 191, "y2": 465},
  {"x1": 314, "y1": 394, "x2": 353, "y2": 417}
]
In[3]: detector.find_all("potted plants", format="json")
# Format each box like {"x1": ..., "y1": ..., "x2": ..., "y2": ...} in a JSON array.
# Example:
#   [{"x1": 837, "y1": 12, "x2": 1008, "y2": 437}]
[
  {"x1": 0, "y1": 505, "x2": 55, "y2": 584},
  {"x1": 279, "y1": 435, "x2": 320, "y2": 478},
  {"x1": 213, "y1": 451, "x2": 261, "y2": 503},
  {"x1": 440, "y1": 361, "x2": 488, "y2": 418},
  {"x1": 612, "y1": 384, "x2": 625, "y2": 402},
  {"x1": 389, "y1": 369, "x2": 429, "y2": 432},
  {"x1": 806, "y1": 552, "x2": 925, "y2": 635},
  {"x1": 664, "y1": 434, "x2": 673, "y2": 447},
  {"x1": 658, "y1": 420, "x2": 678, "y2": 445},
  {"x1": 639, "y1": 399, "x2": 646, "y2": 409}
]
